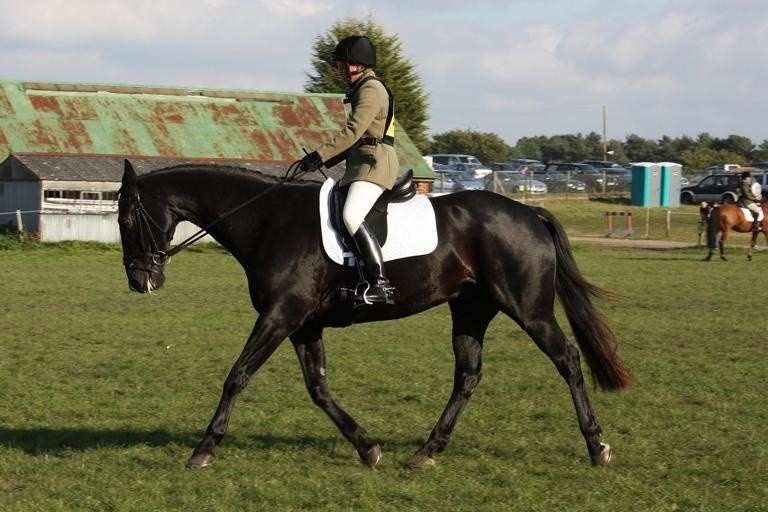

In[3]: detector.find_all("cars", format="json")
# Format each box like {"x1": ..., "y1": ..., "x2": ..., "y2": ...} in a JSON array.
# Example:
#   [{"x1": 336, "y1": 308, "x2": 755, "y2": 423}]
[{"x1": 430, "y1": 153, "x2": 630, "y2": 194}]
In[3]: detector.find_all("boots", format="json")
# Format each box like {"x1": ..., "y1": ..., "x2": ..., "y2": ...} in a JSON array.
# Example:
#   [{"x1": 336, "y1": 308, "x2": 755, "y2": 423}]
[{"x1": 752, "y1": 212, "x2": 763, "y2": 232}]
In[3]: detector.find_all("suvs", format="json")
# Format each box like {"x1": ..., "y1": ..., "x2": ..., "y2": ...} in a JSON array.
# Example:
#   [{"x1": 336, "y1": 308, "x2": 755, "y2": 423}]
[{"x1": 680, "y1": 172, "x2": 768, "y2": 204}]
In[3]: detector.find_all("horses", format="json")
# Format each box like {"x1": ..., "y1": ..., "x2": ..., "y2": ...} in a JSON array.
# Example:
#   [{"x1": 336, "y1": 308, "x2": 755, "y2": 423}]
[
  {"x1": 114, "y1": 155, "x2": 632, "y2": 472},
  {"x1": 702, "y1": 199, "x2": 768, "y2": 261}
]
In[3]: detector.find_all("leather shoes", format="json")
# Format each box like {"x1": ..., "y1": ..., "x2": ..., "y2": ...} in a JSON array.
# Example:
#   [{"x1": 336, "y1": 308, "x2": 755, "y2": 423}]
[{"x1": 350, "y1": 220, "x2": 396, "y2": 303}]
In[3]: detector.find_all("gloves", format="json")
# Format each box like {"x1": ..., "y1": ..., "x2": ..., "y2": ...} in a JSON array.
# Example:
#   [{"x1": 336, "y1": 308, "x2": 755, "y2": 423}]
[{"x1": 299, "y1": 151, "x2": 324, "y2": 174}]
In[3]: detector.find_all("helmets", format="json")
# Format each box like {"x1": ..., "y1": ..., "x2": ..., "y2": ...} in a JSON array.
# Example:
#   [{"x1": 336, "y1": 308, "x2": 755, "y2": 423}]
[
  {"x1": 741, "y1": 171, "x2": 751, "y2": 180},
  {"x1": 330, "y1": 36, "x2": 378, "y2": 68}
]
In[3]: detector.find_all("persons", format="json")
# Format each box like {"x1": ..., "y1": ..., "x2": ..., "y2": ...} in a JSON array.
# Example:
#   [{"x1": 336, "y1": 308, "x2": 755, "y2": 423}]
[
  {"x1": 699, "y1": 200, "x2": 719, "y2": 250},
  {"x1": 707, "y1": 200, "x2": 719, "y2": 217},
  {"x1": 736, "y1": 170, "x2": 763, "y2": 233},
  {"x1": 300, "y1": 34, "x2": 400, "y2": 309}
]
